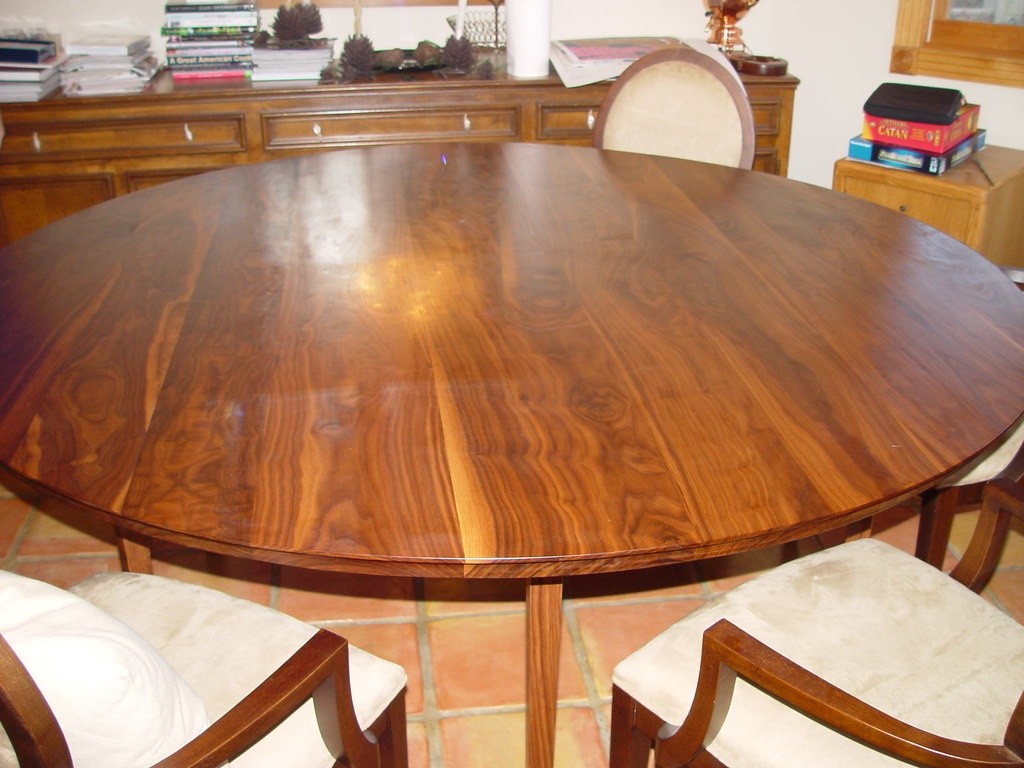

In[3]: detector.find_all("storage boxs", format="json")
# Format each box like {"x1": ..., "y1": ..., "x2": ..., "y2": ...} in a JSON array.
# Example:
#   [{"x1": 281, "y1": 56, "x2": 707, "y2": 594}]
[{"x1": 847, "y1": 103, "x2": 987, "y2": 178}]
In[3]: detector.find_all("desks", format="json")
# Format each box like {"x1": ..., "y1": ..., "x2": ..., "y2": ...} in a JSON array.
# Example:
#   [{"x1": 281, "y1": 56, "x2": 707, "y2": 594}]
[{"x1": 1, "y1": 143, "x2": 1024, "y2": 768}]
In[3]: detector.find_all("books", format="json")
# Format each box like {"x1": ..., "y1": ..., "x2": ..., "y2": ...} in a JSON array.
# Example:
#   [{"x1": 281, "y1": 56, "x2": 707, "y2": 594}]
[
  {"x1": 1, "y1": 1, "x2": 339, "y2": 104},
  {"x1": 548, "y1": 36, "x2": 702, "y2": 88}
]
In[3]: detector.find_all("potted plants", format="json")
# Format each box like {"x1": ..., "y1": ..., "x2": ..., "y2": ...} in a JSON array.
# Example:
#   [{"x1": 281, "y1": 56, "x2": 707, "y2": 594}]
[
  {"x1": 318, "y1": 34, "x2": 507, "y2": 86},
  {"x1": 250, "y1": 1, "x2": 337, "y2": 81}
]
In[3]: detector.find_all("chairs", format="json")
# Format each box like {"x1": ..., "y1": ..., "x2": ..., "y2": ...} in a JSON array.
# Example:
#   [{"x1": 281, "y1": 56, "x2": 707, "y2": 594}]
[
  {"x1": 591, "y1": 49, "x2": 756, "y2": 171},
  {"x1": 1, "y1": 569, "x2": 409, "y2": 768},
  {"x1": 609, "y1": 537, "x2": 1024, "y2": 768}
]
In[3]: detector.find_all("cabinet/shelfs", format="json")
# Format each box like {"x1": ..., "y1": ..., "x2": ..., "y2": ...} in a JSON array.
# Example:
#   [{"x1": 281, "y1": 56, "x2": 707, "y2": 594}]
[
  {"x1": 831, "y1": 145, "x2": 1024, "y2": 271},
  {"x1": 1, "y1": 49, "x2": 800, "y2": 249}
]
